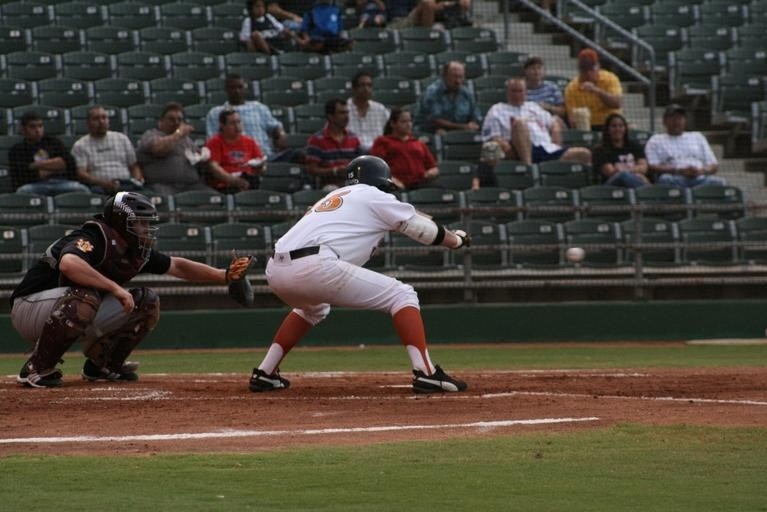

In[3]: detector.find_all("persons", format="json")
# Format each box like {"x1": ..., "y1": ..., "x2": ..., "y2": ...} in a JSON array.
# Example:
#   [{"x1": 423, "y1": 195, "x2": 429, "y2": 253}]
[
  {"x1": 10, "y1": 192, "x2": 249, "y2": 388},
  {"x1": 249, "y1": 155, "x2": 472, "y2": 394}
]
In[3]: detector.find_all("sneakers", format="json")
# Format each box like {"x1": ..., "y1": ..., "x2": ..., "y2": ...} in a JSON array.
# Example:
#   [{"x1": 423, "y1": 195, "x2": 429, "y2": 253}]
[
  {"x1": 81, "y1": 359, "x2": 139, "y2": 382},
  {"x1": 249, "y1": 368, "x2": 289, "y2": 392},
  {"x1": 16, "y1": 361, "x2": 65, "y2": 388},
  {"x1": 413, "y1": 364, "x2": 467, "y2": 393}
]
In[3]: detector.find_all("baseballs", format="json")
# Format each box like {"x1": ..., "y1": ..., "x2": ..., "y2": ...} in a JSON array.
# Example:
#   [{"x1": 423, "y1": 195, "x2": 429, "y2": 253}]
[{"x1": 567, "y1": 248, "x2": 585, "y2": 262}]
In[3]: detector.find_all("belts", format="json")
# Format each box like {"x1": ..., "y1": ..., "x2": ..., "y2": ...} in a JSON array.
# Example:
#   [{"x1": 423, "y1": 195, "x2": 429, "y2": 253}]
[{"x1": 271, "y1": 246, "x2": 319, "y2": 259}]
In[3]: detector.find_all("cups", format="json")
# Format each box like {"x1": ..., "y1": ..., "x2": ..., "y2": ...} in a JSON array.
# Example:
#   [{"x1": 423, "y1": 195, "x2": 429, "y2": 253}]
[
  {"x1": 571, "y1": 107, "x2": 592, "y2": 141},
  {"x1": 480, "y1": 142, "x2": 497, "y2": 167}
]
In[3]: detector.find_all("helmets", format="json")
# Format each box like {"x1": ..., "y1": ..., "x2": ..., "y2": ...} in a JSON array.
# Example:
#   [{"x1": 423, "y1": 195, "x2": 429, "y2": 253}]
[
  {"x1": 347, "y1": 155, "x2": 405, "y2": 188},
  {"x1": 104, "y1": 191, "x2": 160, "y2": 272}
]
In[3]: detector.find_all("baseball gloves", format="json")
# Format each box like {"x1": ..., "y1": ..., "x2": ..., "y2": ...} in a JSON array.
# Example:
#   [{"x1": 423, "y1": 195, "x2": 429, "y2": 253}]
[{"x1": 226, "y1": 249, "x2": 257, "y2": 307}]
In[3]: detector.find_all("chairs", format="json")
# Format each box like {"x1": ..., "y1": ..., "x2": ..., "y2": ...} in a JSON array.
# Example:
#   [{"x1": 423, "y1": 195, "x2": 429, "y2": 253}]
[{"x1": 1, "y1": 1, "x2": 765, "y2": 291}]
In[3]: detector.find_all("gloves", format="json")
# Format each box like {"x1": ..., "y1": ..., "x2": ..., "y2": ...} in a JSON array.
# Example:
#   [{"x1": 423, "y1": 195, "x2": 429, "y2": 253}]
[{"x1": 452, "y1": 229, "x2": 470, "y2": 249}]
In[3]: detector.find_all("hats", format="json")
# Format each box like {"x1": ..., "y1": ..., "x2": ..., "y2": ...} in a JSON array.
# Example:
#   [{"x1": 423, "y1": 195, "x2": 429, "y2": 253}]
[{"x1": 578, "y1": 49, "x2": 597, "y2": 69}]
